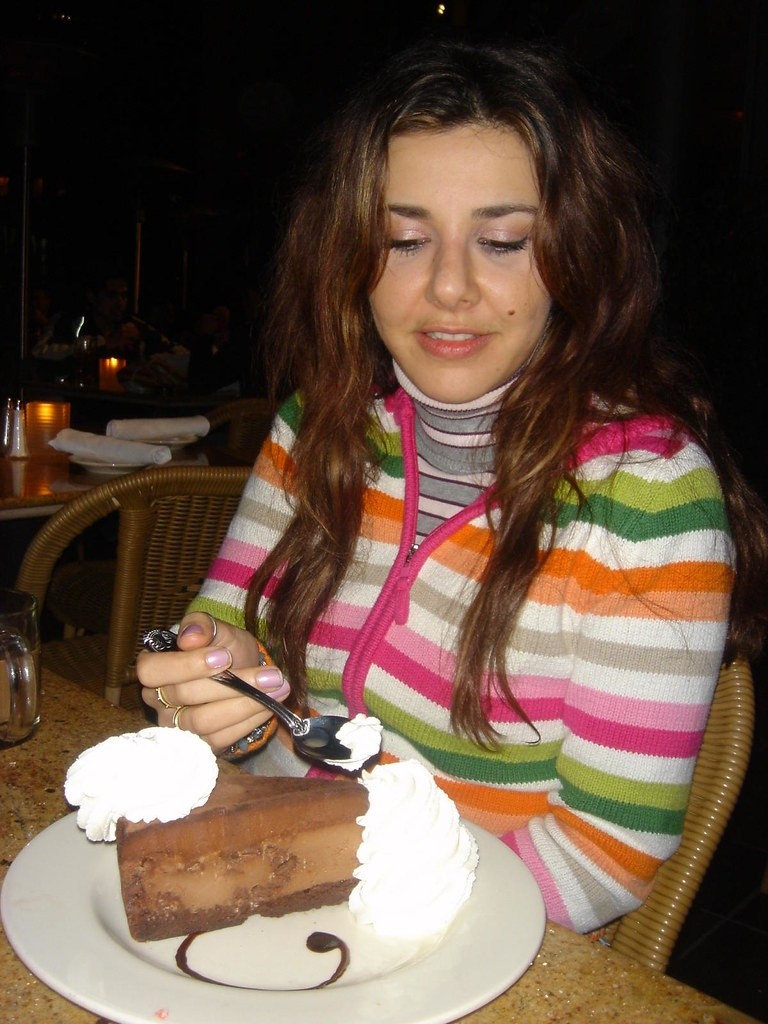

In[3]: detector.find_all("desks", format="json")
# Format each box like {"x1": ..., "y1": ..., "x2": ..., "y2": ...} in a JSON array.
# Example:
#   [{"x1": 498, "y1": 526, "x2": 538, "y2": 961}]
[
  {"x1": 0, "y1": 651, "x2": 759, "y2": 1024},
  {"x1": 0, "y1": 419, "x2": 251, "y2": 521}
]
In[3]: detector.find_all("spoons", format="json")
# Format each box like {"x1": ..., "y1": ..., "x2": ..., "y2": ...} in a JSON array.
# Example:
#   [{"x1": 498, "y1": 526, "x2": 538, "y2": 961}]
[{"x1": 146, "y1": 627, "x2": 368, "y2": 763}]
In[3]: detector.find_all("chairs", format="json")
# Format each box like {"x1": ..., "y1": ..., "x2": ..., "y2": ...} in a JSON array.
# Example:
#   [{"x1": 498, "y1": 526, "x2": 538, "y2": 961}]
[
  {"x1": 205, "y1": 398, "x2": 286, "y2": 465},
  {"x1": 588, "y1": 625, "x2": 754, "y2": 974},
  {"x1": 12, "y1": 467, "x2": 254, "y2": 724}
]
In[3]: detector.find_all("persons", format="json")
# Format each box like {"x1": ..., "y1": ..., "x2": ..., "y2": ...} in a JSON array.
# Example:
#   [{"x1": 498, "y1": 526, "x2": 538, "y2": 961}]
[
  {"x1": 25, "y1": 266, "x2": 249, "y2": 395},
  {"x1": 137, "y1": 29, "x2": 768, "y2": 932}
]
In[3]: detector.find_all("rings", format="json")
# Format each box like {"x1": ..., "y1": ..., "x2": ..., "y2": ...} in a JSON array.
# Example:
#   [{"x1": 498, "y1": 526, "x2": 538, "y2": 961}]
[
  {"x1": 156, "y1": 687, "x2": 188, "y2": 729},
  {"x1": 207, "y1": 614, "x2": 216, "y2": 640}
]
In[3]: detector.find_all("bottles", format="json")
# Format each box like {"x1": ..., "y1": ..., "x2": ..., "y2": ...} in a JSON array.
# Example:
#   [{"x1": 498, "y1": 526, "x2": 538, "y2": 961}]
[
  {"x1": 0, "y1": 397, "x2": 16, "y2": 454},
  {"x1": 7, "y1": 400, "x2": 30, "y2": 459}
]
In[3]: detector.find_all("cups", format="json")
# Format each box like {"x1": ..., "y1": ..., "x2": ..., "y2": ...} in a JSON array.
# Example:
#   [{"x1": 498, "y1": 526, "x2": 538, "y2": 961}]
[{"x1": 0, "y1": 589, "x2": 41, "y2": 745}]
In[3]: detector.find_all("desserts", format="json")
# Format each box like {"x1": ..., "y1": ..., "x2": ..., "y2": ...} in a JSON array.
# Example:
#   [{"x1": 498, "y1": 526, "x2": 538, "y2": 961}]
[{"x1": 62, "y1": 724, "x2": 371, "y2": 944}]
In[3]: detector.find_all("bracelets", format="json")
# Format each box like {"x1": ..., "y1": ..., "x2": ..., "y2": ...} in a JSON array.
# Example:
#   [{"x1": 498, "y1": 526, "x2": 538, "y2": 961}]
[{"x1": 221, "y1": 651, "x2": 271, "y2": 761}]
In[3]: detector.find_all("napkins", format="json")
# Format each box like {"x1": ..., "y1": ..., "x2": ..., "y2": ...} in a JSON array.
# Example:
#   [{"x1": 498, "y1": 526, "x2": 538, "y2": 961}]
[
  {"x1": 48, "y1": 428, "x2": 172, "y2": 467},
  {"x1": 106, "y1": 416, "x2": 210, "y2": 439}
]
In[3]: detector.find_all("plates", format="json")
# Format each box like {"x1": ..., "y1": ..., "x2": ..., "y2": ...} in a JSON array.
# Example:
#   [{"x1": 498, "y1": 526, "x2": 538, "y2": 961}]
[
  {"x1": 130, "y1": 435, "x2": 198, "y2": 452},
  {"x1": 0, "y1": 807, "x2": 547, "y2": 1021},
  {"x1": 69, "y1": 455, "x2": 143, "y2": 474}
]
